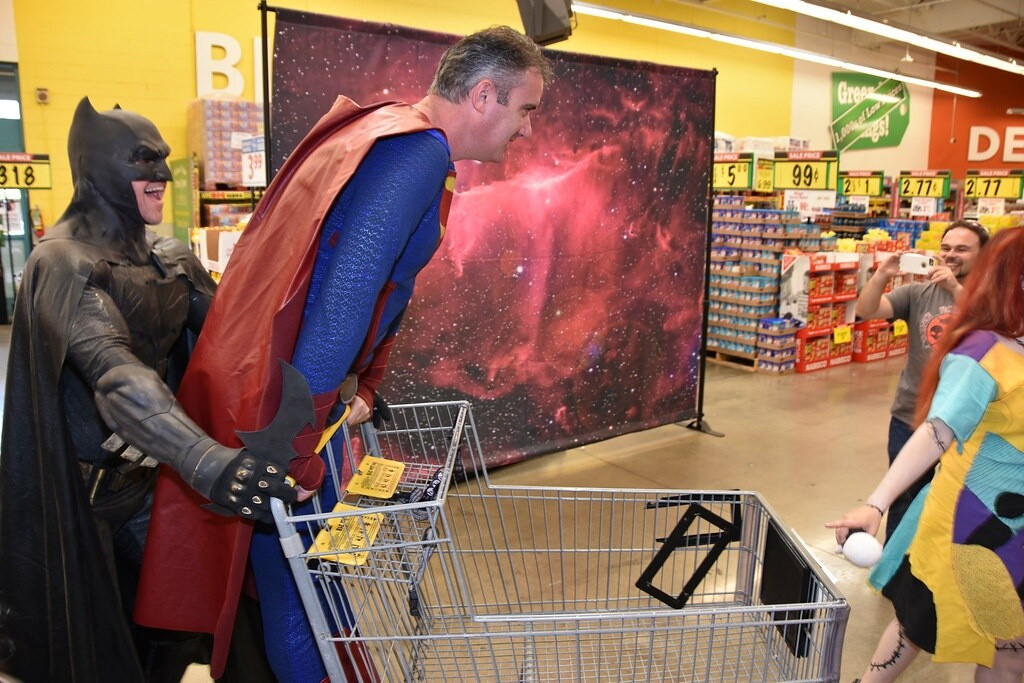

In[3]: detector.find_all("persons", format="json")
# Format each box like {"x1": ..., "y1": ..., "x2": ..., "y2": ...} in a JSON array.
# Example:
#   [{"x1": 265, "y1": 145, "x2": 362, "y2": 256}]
[
  {"x1": 132, "y1": 23, "x2": 550, "y2": 683},
  {"x1": 2, "y1": 97, "x2": 394, "y2": 682},
  {"x1": 825, "y1": 224, "x2": 1024, "y2": 682},
  {"x1": 856, "y1": 221, "x2": 991, "y2": 545}
]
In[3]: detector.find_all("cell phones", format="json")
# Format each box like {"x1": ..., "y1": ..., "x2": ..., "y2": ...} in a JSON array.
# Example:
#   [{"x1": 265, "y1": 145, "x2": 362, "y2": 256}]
[{"x1": 898, "y1": 253, "x2": 935, "y2": 275}]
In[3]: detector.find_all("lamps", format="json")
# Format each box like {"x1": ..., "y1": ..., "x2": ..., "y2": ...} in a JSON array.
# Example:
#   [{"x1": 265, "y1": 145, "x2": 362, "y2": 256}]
[
  {"x1": 759, "y1": 0, "x2": 1024, "y2": 75},
  {"x1": 568, "y1": 0, "x2": 984, "y2": 99}
]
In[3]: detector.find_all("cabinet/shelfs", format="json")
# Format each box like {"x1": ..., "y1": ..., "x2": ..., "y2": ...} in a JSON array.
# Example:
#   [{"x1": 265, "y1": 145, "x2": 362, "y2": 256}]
[
  {"x1": 187, "y1": 149, "x2": 267, "y2": 285},
  {"x1": 704, "y1": 150, "x2": 1022, "y2": 378}
]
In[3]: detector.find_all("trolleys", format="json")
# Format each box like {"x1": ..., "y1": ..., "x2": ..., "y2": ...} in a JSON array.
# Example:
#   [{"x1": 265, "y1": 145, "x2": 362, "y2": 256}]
[{"x1": 270, "y1": 400, "x2": 852, "y2": 683}]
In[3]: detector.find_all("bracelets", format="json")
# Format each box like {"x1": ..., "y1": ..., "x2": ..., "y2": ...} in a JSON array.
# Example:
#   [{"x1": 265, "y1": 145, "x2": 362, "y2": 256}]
[{"x1": 864, "y1": 503, "x2": 884, "y2": 518}]
